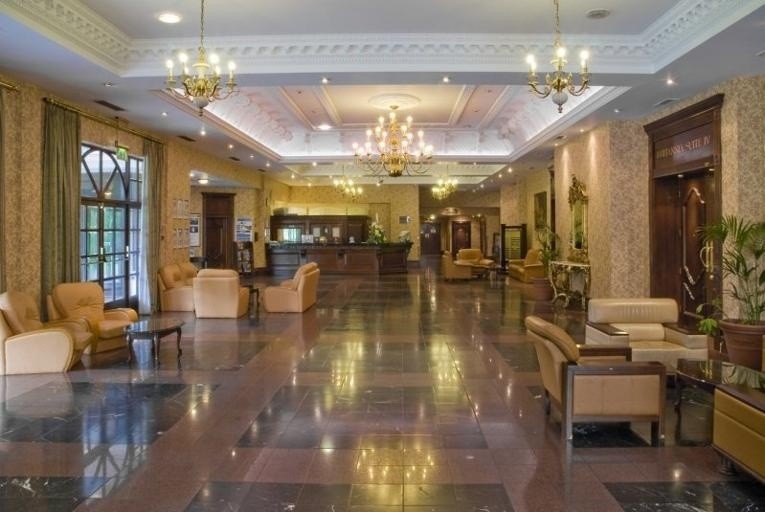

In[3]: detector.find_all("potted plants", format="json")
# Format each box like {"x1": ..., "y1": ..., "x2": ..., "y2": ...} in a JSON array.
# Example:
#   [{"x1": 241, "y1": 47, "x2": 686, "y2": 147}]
[{"x1": 696, "y1": 216, "x2": 764, "y2": 371}]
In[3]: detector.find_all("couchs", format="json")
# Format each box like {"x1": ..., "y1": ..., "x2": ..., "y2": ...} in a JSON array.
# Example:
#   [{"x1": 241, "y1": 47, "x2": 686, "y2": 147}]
[
  {"x1": 46, "y1": 281, "x2": 139, "y2": 354},
  {"x1": 0, "y1": 290, "x2": 95, "y2": 374},
  {"x1": 584, "y1": 297, "x2": 709, "y2": 390},
  {"x1": 157, "y1": 263, "x2": 197, "y2": 313}
]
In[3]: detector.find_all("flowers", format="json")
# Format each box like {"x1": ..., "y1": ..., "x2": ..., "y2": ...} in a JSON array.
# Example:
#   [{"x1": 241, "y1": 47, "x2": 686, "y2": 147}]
[{"x1": 371, "y1": 221, "x2": 385, "y2": 244}]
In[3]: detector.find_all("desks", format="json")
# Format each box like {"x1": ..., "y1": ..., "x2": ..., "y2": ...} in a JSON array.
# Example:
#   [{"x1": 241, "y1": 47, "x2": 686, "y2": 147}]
[{"x1": 548, "y1": 260, "x2": 589, "y2": 308}]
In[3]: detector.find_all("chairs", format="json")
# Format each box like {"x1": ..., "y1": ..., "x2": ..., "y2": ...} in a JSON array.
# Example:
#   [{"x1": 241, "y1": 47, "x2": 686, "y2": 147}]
[
  {"x1": 525, "y1": 315, "x2": 667, "y2": 448},
  {"x1": 456, "y1": 248, "x2": 494, "y2": 279},
  {"x1": 263, "y1": 262, "x2": 321, "y2": 312},
  {"x1": 442, "y1": 250, "x2": 473, "y2": 281},
  {"x1": 193, "y1": 268, "x2": 249, "y2": 318},
  {"x1": 508, "y1": 248, "x2": 545, "y2": 282},
  {"x1": 710, "y1": 385, "x2": 765, "y2": 484}
]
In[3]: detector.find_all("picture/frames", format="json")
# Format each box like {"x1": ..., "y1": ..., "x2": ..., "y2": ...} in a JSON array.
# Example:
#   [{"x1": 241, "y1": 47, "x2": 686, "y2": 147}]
[
  {"x1": 569, "y1": 174, "x2": 589, "y2": 260},
  {"x1": 535, "y1": 190, "x2": 548, "y2": 230}
]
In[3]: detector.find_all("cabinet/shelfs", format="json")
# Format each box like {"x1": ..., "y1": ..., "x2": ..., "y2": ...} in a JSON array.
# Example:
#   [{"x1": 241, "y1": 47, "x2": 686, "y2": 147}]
[
  {"x1": 271, "y1": 214, "x2": 371, "y2": 245},
  {"x1": 502, "y1": 222, "x2": 528, "y2": 267}
]
